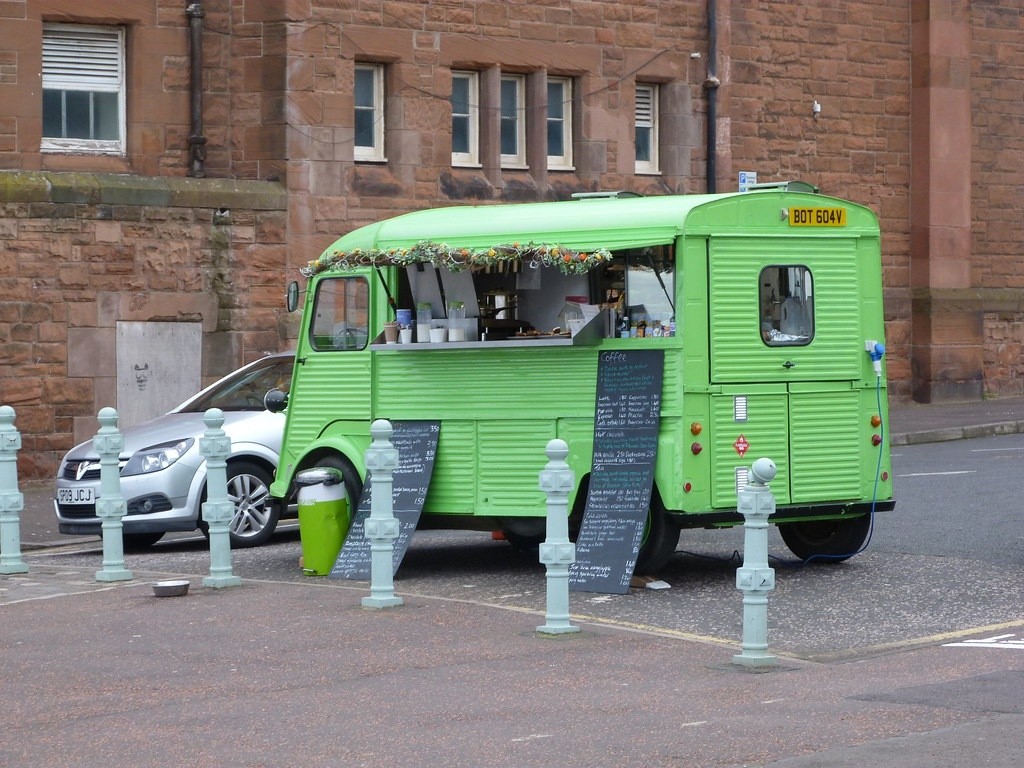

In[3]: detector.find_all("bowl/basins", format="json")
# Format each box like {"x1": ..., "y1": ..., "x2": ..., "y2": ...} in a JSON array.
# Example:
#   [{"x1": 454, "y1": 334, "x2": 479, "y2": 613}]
[{"x1": 150, "y1": 580, "x2": 189, "y2": 595}]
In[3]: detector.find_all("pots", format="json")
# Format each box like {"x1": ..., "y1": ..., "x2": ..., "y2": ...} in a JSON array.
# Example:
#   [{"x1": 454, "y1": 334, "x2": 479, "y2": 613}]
[
  {"x1": 480, "y1": 306, "x2": 517, "y2": 316},
  {"x1": 485, "y1": 286, "x2": 522, "y2": 320}
]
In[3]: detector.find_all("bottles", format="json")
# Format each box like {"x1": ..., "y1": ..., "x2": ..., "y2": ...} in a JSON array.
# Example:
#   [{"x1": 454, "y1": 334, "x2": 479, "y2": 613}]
[
  {"x1": 449, "y1": 301, "x2": 465, "y2": 342},
  {"x1": 417, "y1": 303, "x2": 431, "y2": 343},
  {"x1": 781, "y1": 296, "x2": 812, "y2": 337},
  {"x1": 620, "y1": 316, "x2": 675, "y2": 338},
  {"x1": 761, "y1": 283, "x2": 773, "y2": 313}
]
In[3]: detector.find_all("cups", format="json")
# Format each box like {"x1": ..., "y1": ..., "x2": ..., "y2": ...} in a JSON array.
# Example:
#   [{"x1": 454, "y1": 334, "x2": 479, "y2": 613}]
[
  {"x1": 400, "y1": 330, "x2": 412, "y2": 343},
  {"x1": 396, "y1": 309, "x2": 411, "y2": 342},
  {"x1": 383, "y1": 326, "x2": 398, "y2": 344},
  {"x1": 429, "y1": 328, "x2": 446, "y2": 342}
]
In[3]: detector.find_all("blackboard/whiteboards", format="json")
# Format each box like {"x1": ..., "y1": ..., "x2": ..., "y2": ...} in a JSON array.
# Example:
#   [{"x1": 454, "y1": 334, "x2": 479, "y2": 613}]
[
  {"x1": 566, "y1": 350, "x2": 666, "y2": 595},
  {"x1": 328, "y1": 420, "x2": 442, "y2": 578}
]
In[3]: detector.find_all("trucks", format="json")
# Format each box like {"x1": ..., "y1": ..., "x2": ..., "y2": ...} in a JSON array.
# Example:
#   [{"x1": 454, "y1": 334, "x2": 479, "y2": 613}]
[{"x1": 262, "y1": 177, "x2": 899, "y2": 580}]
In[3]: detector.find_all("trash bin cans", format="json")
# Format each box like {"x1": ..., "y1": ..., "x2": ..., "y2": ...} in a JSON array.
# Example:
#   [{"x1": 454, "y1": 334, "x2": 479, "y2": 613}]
[{"x1": 294, "y1": 467, "x2": 349, "y2": 577}]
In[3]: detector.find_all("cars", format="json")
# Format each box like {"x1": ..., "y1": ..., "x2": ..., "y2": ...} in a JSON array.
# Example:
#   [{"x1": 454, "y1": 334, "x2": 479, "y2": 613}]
[{"x1": 51, "y1": 347, "x2": 299, "y2": 551}]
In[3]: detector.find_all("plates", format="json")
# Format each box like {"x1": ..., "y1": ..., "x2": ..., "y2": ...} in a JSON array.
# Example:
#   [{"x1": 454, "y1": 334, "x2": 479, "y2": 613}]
[{"x1": 507, "y1": 334, "x2": 570, "y2": 340}]
